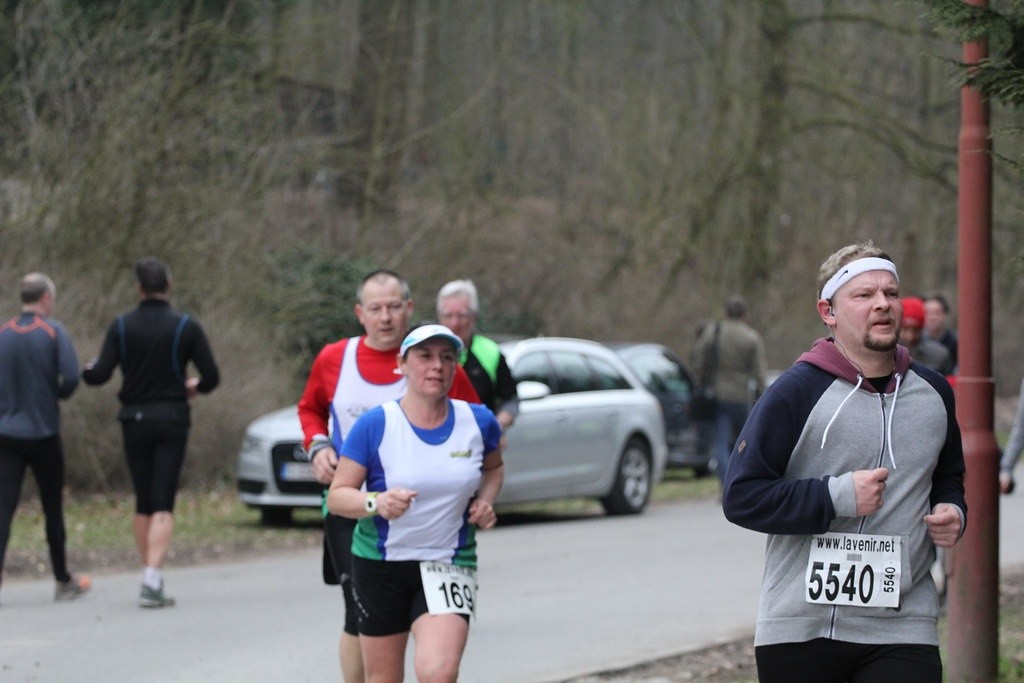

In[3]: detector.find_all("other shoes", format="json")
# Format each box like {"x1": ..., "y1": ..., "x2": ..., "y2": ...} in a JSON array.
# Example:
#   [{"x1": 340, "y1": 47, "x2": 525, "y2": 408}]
[
  {"x1": 140, "y1": 584, "x2": 175, "y2": 608},
  {"x1": 57, "y1": 575, "x2": 89, "y2": 601}
]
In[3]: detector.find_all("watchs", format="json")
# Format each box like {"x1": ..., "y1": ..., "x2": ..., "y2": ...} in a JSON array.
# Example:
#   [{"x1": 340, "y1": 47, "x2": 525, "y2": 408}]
[{"x1": 364, "y1": 491, "x2": 379, "y2": 516}]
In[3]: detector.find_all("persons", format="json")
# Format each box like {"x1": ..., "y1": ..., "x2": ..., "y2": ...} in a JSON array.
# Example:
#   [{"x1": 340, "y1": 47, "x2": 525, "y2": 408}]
[
  {"x1": 686, "y1": 290, "x2": 769, "y2": 481},
  {"x1": 325, "y1": 323, "x2": 504, "y2": 683},
  {"x1": 997, "y1": 376, "x2": 1023, "y2": 494},
  {"x1": 79, "y1": 256, "x2": 221, "y2": 609},
  {"x1": 893, "y1": 294, "x2": 958, "y2": 375},
  {"x1": 719, "y1": 242, "x2": 968, "y2": 683},
  {"x1": 0, "y1": 271, "x2": 90, "y2": 602},
  {"x1": 299, "y1": 270, "x2": 483, "y2": 683},
  {"x1": 434, "y1": 279, "x2": 520, "y2": 434}
]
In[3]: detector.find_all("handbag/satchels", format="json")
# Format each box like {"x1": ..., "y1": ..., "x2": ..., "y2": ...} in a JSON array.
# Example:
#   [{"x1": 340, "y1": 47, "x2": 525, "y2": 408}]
[{"x1": 692, "y1": 322, "x2": 720, "y2": 420}]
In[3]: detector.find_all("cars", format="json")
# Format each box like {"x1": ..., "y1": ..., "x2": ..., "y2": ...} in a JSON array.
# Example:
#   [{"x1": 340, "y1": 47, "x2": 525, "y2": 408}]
[
  {"x1": 236, "y1": 334, "x2": 666, "y2": 529},
  {"x1": 587, "y1": 339, "x2": 719, "y2": 480}
]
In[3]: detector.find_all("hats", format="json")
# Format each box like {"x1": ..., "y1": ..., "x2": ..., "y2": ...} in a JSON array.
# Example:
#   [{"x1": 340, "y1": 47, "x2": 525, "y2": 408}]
[
  {"x1": 400, "y1": 324, "x2": 464, "y2": 360},
  {"x1": 901, "y1": 297, "x2": 924, "y2": 328}
]
